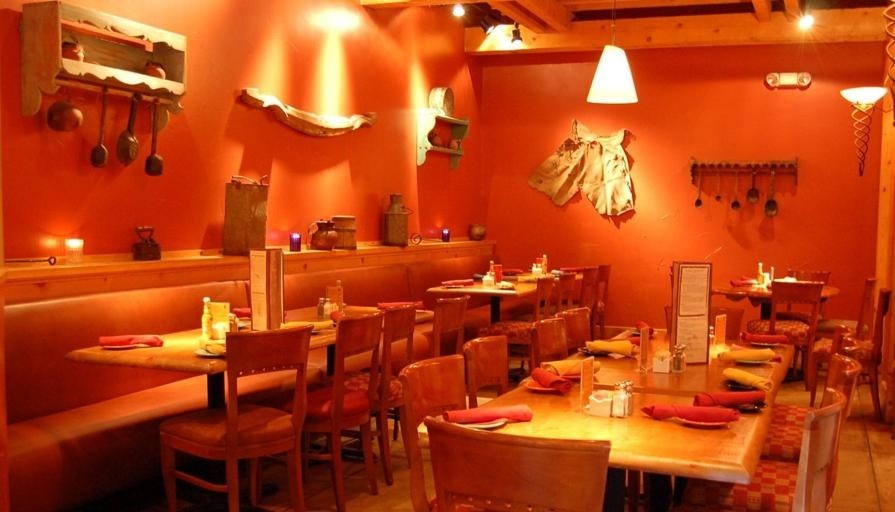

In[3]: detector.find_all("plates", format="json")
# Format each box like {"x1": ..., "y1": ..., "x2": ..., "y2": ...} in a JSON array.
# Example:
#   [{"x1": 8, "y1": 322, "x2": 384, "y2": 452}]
[
  {"x1": 676, "y1": 416, "x2": 729, "y2": 429},
  {"x1": 738, "y1": 359, "x2": 771, "y2": 366},
  {"x1": 101, "y1": 344, "x2": 148, "y2": 350},
  {"x1": 460, "y1": 421, "x2": 508, "y2": 431},
  {"x1": 578, "y1": 346, "x2": 609, "y2": 357},
  {"x1": 195, "y1": 349, "x2": 228, "y2": 358},
  {"x1": 523, "y1": 374, "x2": 580, "y2": 391}
]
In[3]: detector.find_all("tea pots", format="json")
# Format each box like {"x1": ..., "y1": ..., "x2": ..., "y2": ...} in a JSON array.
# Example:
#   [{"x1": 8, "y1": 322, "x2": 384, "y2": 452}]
[
  {"x1": 130, "y1": 226, "x2": 161, "y2": 261},
  {"x1": 308, "y1": 219, "x2": 338, "y2": 250}
]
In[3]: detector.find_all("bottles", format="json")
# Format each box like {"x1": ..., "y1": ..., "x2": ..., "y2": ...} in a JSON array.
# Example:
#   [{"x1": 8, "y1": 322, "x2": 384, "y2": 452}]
[
  {"x1": 671, "y1": 342, "x2": 688, "y2": 373},
  {"x1": 199, "y1": 306, "x2": 239, "y2": 338},
  {"x1": 532, "y1": 263, "x2": 543, "y2": 274},
  {"x1": 484, "y1": 272, "x2": 496, "y2": 286},
  {"x1": 316, "y1": 298, "x2": 332, "y2": 319},
  {"x1": 757, "y1": 272, "x2": 770, "y2": 287},
  {"x1": 610, "y1": 378, "x2": 635, "y2": 419}
]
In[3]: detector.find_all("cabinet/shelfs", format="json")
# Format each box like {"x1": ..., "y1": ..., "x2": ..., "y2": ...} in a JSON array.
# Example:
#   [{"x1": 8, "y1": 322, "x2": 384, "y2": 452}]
[
  {"x1": 15, "y1": 3, "x2": 189, "y2": 134},
  {"x1": 417, "y1": 107, "x2": 467, "y2": 175}
]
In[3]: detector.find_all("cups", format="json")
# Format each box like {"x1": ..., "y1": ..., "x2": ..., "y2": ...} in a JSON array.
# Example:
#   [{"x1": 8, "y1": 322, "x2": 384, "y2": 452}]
[
  {"x1": 288, "y1": 233, "x2": 302, "y2": 252},
  {"x1": 441, "y1": 226, "x2": 450, "y2": 241},
  {"x1": 66, "y1": 237, "x2": 84, "y2": 264}
]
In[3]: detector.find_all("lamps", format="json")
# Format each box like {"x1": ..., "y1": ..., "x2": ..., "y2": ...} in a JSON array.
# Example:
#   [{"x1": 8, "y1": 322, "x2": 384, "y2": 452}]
[
  {"x1": 478, "y1": 16, "x2": 530, "y2": 53},
  {"x1": 585, "y1": 2, "x2": 641, "y2": 106},
  {"x1": 836, "y1": 84, "x2": 895, "y2": 176}
]
